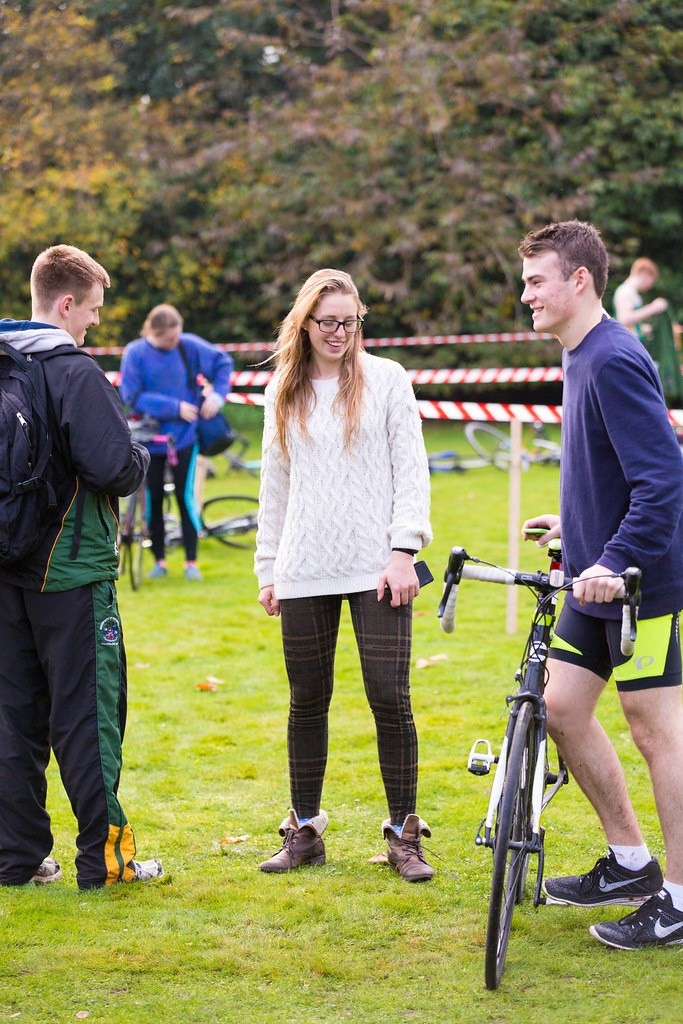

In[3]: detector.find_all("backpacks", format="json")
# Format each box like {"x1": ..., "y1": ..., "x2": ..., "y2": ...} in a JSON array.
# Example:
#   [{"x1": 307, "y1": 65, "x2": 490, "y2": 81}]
[{"x1": 0, "y1": 341, "x2": 98, "y2": 564}]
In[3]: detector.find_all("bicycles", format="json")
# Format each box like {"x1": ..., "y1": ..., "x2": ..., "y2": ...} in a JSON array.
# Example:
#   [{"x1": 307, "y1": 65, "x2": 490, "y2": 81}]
[
  {"x1": 153, "y1": 496, "x2": 259, "y2": 549},
  {"x1": 119, "y1": 418, "x2": 158, "y2": 592},
  {"x1": 435, "y1": 538, "x2": 643, "y2": 991},
  {"x1": 428, "y1": 421, "x2": 562, "y2": 471}
]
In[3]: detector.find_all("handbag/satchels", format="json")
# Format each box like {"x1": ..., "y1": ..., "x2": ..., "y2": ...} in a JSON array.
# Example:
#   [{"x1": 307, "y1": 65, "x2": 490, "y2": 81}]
[{"x1": 178, "y1": 341, "x2": 234, "y2": 457}]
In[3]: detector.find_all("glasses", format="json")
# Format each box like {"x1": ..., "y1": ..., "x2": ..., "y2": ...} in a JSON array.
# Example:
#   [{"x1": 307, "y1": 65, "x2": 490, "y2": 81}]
[{"x1": 307, "y1": 313, "x2": 364, "y2": 333}]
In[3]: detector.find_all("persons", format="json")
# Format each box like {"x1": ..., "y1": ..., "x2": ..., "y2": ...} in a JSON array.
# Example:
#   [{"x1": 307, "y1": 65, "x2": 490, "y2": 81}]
[
  {"x1": 119, "y1": 304, "x2": 234, "y2": 580},
  {"x1": 0, "y1": 244, "x2": 171, "y2": 889},
  {"x1": 613, "y1": 257, "x2": 668, "y2": 343},
  {"x1": 252, "y1": 269, "x2": 434, "y2": 883},
  {"x1": 518, "y1": 216, "x2": 683, "y2": 950}
]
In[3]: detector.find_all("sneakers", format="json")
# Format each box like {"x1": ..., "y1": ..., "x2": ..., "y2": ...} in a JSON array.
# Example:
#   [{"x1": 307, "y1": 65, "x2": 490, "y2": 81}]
[
  {"x1": 542, "y1": 846, "x2": 663, "y2": 907},
  {"x1": 149, "y1": 559, "x2": 168, "y2": 579},
  {"x1": 183, "y1": 560, "x2": 202, "y2": 581},
  {"x1": 117, "y1": 860, "x2": 164, "y2": 883},
  {"x1": 28, "y1": 856, "x2": 61, "y2": 883},
  {"x1": 588, "y1": 887, "x2": 683, "y2": 951}
]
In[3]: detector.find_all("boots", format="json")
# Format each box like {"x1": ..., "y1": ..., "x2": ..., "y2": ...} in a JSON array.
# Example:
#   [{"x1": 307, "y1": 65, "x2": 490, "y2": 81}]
[
  {"x1": 259, "y1": 808, "x2": 329, "y2": 872},
  {"x1": 382, "y1": 813, "x2": 443, "y2": 881}
]
